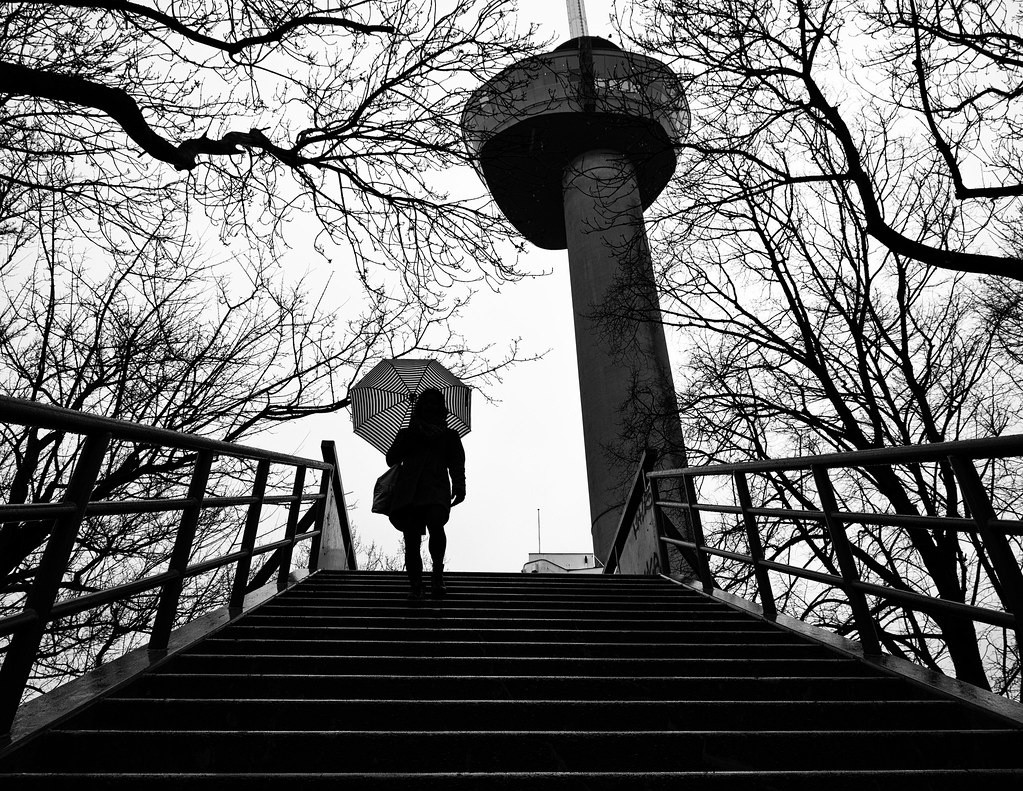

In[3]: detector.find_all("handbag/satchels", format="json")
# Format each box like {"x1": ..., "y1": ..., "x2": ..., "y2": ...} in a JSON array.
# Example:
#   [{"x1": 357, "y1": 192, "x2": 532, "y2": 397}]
[{"x1": 372, "y1": 462, "x2": 404, "y2": 515}]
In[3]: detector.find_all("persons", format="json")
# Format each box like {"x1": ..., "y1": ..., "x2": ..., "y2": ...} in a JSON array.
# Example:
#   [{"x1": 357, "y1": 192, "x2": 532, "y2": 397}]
[{"x1": 387, "y1": 389, "x2": 466, "y2": 603}]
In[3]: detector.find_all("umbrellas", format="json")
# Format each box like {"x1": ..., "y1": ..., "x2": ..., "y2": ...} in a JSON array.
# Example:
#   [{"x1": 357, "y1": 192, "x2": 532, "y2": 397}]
[{"x1": 350, "y1": 357, "x2": 472, "y2": 454}]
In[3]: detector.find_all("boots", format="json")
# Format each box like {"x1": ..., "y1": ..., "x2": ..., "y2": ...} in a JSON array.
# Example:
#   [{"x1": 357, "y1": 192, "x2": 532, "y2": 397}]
[
  {"x1": 407, "y1": 573, "x2": 426, "y2": 604},
  {"x1": 429, "y1": 562, "x2": 446, "y2": 590}
]
